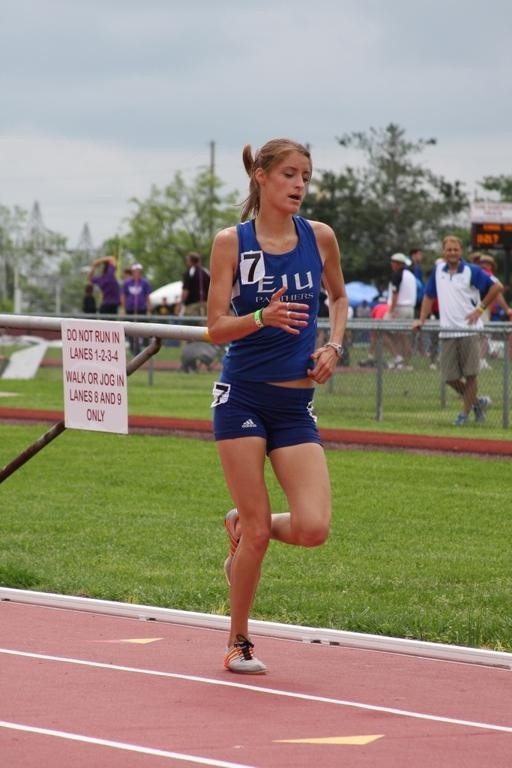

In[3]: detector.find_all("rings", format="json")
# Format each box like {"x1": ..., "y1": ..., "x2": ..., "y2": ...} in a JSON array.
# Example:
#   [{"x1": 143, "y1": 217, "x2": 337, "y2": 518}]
[
  {"x1": 287, "y1": 311, "x2": 291, "y2": 318},
  {"x1": 287, "y1": 302, "x2": 290, "y2": 310}
]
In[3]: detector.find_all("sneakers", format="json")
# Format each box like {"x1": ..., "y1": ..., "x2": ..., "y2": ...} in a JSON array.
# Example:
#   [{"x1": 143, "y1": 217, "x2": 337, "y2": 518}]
[
  {"x1": 223, "y1": 509, "x2": 240, "y2": 586},
  {"x1": 223, "y1": 633, "x2": 267, "y2": 674},
  {"x1": 472, "y1": 397, "x2": 490, "y2": 423},
  {"x1": 360, "y1": 354, "x2": 439, "y2": 371},
  {"x1": 456, "y1": 414, "x2": 468, "y2": 426}
]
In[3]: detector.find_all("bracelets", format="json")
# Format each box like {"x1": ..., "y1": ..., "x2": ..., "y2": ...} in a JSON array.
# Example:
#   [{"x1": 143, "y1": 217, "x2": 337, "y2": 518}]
[{"x1": 254, "y1": 309, "x2": 265, "y2": 328}]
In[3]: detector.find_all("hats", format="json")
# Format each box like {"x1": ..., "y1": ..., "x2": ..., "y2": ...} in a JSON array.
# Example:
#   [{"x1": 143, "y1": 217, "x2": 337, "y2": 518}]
[{"x1": 389, "y1": 252, "x2": 412, "y2": 267}]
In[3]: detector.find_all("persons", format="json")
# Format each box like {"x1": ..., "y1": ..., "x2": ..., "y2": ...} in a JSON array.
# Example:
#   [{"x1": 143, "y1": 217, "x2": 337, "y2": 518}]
[
  {"x1": 83, "y1": 252, "x2": 224, "y2": 371},
  {"x1": 315, "y1": 234, "x2": 512, "y2": 427},
  {"x1": 207, "y1": 138, "x2": 348, "y2": 674}
]
